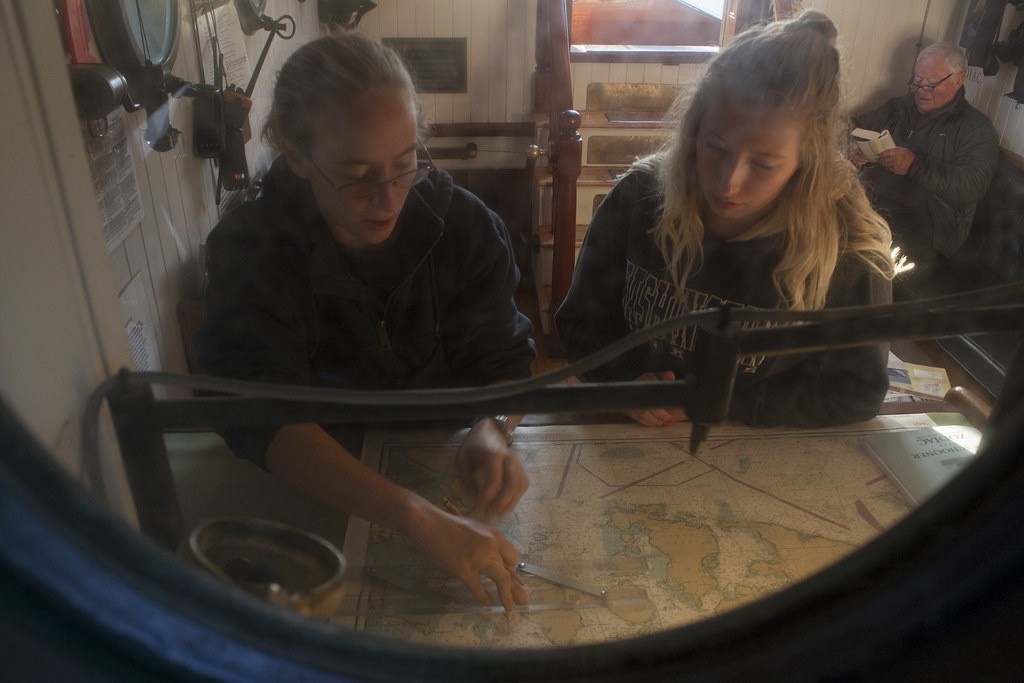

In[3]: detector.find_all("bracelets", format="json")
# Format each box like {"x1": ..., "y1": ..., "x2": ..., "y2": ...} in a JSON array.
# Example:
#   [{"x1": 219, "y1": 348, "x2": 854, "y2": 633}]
[{"x1": 472, "y1": 413, "x2": 513, "y2": 448}]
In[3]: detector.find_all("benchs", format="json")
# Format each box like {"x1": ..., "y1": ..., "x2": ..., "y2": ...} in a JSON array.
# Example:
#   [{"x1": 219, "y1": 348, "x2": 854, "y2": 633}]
[
  {"x1": 853, "y1": 145, "x2": 1023, "y2": 389},
  {"x1": 573, "y1": 108, "x2": 688, "y2": 170}
]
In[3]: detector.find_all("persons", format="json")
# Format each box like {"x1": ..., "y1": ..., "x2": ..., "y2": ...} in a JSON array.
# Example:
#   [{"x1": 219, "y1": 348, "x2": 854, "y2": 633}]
[
  {"x1": 554, "y1": 10, "x2": 915, "y2": 428},
  {"x1": 847, "y1": 44, "x2": 1001, "y2": 294},
  {"x1": 193, "y1": 36, "x2": 537, "y2": 613}
]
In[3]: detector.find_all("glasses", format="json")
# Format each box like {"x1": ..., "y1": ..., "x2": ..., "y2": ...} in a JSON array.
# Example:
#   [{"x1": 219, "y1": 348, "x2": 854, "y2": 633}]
[
  {"x1": 296, "y1": 134, "x2": 436, "y2": 200},
  {"x1": 908, "y1": 72, "x2": 956, "y2": 93}
]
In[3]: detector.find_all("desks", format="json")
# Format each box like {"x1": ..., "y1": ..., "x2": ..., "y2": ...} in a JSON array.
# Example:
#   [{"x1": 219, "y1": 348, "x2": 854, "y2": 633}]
[{"x1": 162, "y1": 378, "x2": 996, "y2": 643}]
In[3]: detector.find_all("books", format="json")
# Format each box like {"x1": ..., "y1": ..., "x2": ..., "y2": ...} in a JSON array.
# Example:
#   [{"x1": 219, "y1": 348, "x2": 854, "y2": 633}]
[{"x1": 851, "y1": 127, "x2": 899, "y2": 165}]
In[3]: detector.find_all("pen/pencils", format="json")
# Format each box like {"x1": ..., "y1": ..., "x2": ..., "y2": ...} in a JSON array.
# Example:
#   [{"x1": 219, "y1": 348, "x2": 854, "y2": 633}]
[{"x1": 440, "y1": 497, "x2": 529, "y2": 591}]
